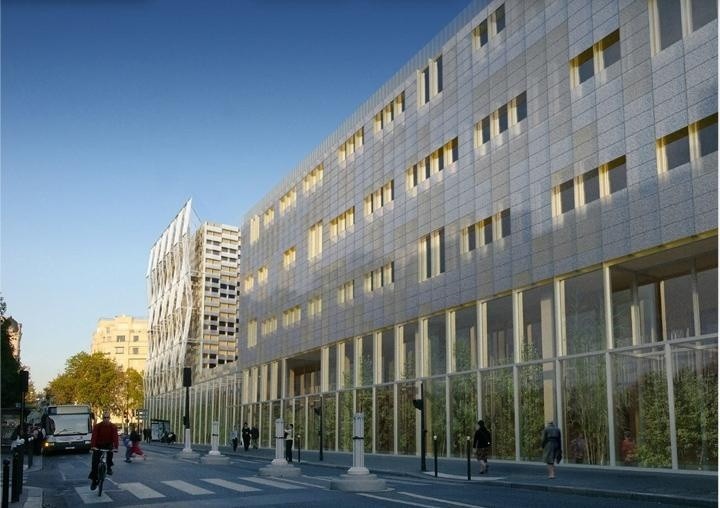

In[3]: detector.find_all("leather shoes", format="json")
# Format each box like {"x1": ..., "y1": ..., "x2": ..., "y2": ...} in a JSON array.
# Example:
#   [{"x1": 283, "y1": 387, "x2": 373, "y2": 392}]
[
  {"x1": 91, "y1": 483, "x2": 97, "y2": 490},
  {"x1": 107, "y1": 468, "x2": 113, "y2": 475},
  {"x1": 485, "y1": 465, "x2": 488, "y2": 473}
]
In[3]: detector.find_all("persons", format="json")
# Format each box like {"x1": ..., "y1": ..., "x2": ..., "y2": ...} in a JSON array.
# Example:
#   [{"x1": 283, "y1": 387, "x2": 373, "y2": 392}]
[
  {"x1": 569, "y1": 432, "x2": 587, "y2": 464},
  {"x1": 285, "y1": 423, "x2": 293, "y2": 463},
  {"x1": 143, "y1": 427, "x2": 177, "y2": 446},
  {"x1": 472, "y1": 420, "x2": 491, "y2": 475},
  {"x1": 88, "y1": 412, "x2": 119, "y2": 491},
  {"x1": 121, "y1": 430, "x2": 133, "y2": 457},
  {"x1": 230, "y1": 424, "x2": 239, "y2": 452},
  {"x1": 541, "y1": 420, "x2": 562, "y2": 480},
  {"x1": 10, "y1": 421, "x2": 46, "y2": 456},
  {"x1": 620, "y1": 430, "x2": 635, "y2": 465},
  {"x1": 123, "y1": 424, "x2": 148, "y2": 464},
  {"x1": 241, "y1": 422, "x2": 251, "y2": 452},
  {"x1": 249, "y1": 426, "x2": 259, "y2": 449}
]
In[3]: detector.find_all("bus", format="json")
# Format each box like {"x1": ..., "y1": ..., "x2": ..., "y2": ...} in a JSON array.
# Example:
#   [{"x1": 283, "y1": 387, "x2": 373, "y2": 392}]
[{"x1": 0, "y1": 404, "x2": 96, "y2": 458}]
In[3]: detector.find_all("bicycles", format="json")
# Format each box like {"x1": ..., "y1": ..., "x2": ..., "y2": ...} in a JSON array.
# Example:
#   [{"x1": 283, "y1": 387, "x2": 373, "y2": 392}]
[{"x1": 89, "y1": 446, "x2": 120, "y2": 496}]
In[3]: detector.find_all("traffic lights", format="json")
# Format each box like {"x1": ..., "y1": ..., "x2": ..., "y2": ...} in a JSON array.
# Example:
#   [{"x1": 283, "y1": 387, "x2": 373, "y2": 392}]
[
  {"x1": 313, "y1": 406, "x2": 322, "y2": 416},
  {"x1": 412, "y1": 398, "x2": 422, "y2": 410}
]
